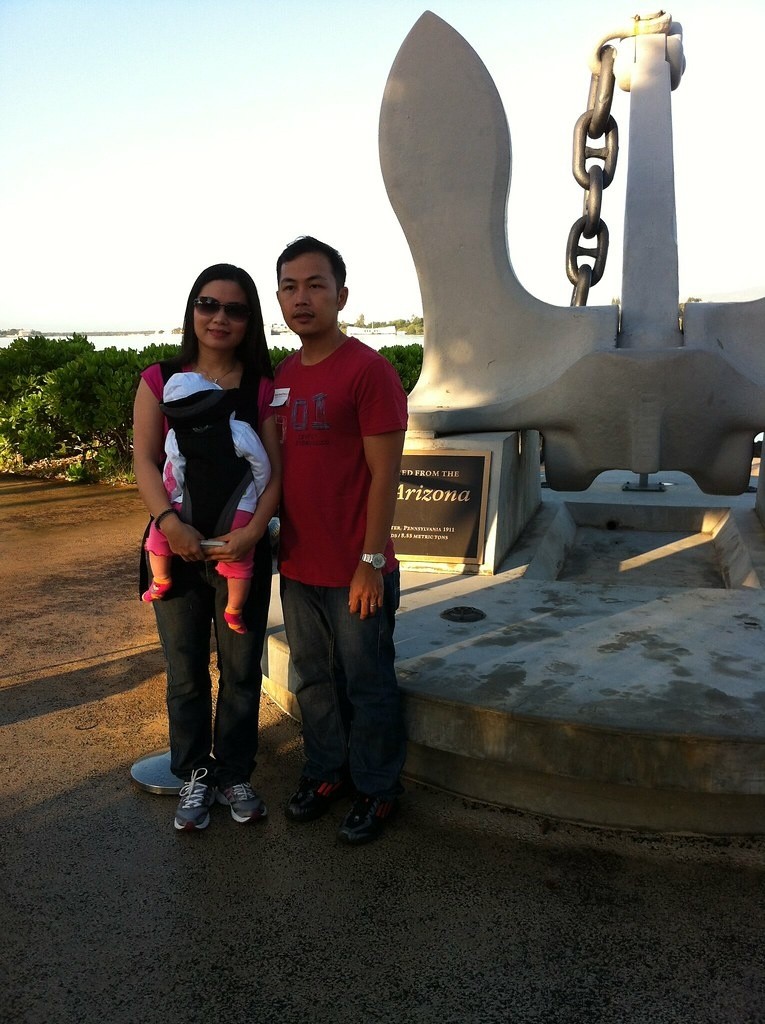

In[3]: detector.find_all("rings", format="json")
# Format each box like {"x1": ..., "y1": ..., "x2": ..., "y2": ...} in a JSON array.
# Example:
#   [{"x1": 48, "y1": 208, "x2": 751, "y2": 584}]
[{"x1": 370, "y1": 604, "x2": 376, "y2": 607}]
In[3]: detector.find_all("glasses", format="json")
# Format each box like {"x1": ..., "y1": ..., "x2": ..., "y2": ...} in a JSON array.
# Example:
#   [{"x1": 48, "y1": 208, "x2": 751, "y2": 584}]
[{"x1": 194, "y1": 296, "x2": 249, "y2": 322}]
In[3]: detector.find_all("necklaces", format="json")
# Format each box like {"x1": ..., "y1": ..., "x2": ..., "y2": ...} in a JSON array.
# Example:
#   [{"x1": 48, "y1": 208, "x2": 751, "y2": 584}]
[{"x1": 195, "y1": 361, "x2": 238, "y2": 384}]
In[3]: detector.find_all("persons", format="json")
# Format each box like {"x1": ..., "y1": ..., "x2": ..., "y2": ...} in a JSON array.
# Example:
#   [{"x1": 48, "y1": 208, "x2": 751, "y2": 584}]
[
  {"x1": 143, "y1": 372, "x2": 272, "y2": 634},
  {"x1": 131, "y1": 263, "x2": 284, "y2": 833},
  {"x1": 271, "y1": 236, "x2": 408, "y2": 847}
]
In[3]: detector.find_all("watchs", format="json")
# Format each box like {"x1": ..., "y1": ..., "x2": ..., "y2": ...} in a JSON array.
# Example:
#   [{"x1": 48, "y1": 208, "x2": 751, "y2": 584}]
[{"x1": 361, "y1": 552, "x2": 387, "y2": 570}]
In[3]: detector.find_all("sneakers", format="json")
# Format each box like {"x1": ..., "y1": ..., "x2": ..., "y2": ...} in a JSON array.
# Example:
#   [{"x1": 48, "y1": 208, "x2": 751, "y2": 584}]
[
  {"x1": 216, "y1": 781, "x2": 268, "y2": 825},
  {"x1": 285, "y1": 774, "x2": 356, "y2": 823},
  {"x1": 336, "y1": 780, "x2": 407, "y2": 845},
  {"x1": 174, "y1": 768, "x2": 216, "y2": 832}
]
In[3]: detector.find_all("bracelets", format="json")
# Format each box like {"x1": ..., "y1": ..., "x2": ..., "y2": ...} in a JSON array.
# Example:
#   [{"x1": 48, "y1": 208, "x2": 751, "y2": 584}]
[{"x1": 155, "y1": 508, "x2": 177, "y2": 528}]
